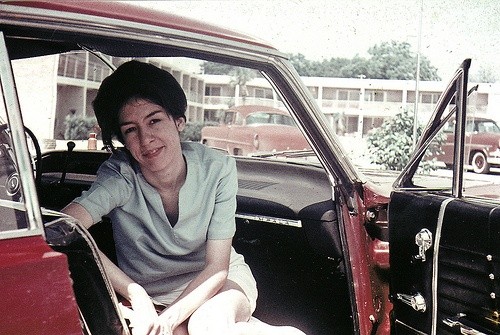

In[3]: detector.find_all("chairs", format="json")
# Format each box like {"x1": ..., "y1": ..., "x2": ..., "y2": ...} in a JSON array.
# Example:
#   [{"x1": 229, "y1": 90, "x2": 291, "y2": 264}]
[{"x1": 1, "y1": 200, "x2": 130, "y2": 335}]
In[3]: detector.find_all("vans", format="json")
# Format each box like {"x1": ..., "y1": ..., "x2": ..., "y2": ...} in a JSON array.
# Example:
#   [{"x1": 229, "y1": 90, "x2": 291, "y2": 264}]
[{"x1": 420, "y1": 117, "x2": 500, "y2": 174}]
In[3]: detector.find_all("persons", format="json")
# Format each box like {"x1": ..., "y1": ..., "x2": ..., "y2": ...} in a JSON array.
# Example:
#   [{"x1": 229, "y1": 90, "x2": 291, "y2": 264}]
[{"x1": 54, "y1": 60, "x2": 305, "y2": 335}]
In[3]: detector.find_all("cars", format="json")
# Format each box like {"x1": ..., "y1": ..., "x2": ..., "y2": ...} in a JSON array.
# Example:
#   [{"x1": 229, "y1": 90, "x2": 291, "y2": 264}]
[
  {"x1": 201, "y1": 105, "x2": 312, "y2": 159},
  {"x1": 0, "y1": 0, "x2": 500, "y2": 335}
]
